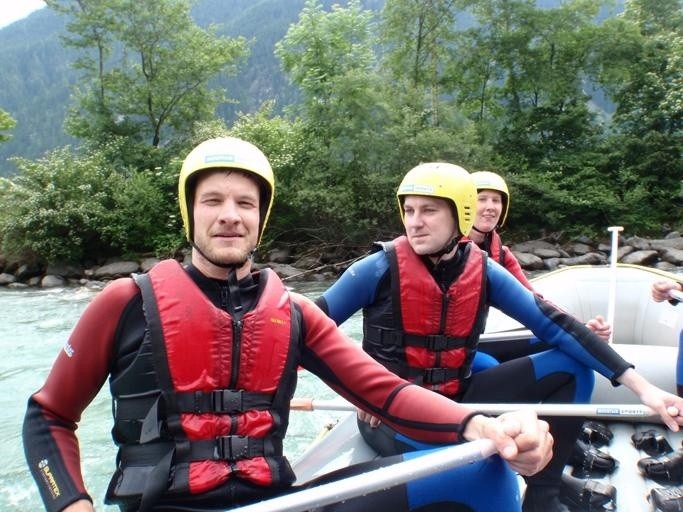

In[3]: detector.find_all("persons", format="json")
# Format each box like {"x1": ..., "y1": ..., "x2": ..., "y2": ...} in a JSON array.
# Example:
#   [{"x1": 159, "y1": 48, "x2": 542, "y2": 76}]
[
  {"x1": 306, "y1": 159, "x2": 682, "y2": 511},
  {"x1": 368, "y1": 167, "x2": 615, "y2": 475},
  {"x1": 630, "y1": 266, "x2": 682, "y2": 511},
  {"x1": 18, "y1": 136, "x2": 560, "y2": 510}
]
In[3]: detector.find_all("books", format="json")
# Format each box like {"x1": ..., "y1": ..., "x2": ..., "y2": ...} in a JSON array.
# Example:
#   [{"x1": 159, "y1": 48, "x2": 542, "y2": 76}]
[{"x1": 665, "y1": 289, "x2": 682, "y2": 304}]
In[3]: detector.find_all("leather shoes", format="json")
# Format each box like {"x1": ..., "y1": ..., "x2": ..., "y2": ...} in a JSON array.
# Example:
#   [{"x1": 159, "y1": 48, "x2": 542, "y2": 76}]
[
  {"x1": 559, "y1": 473, "x2": 617, "y2": 512},
  {"x1": 638, "y1": 448, "x2": 683, "y2": 485},
  {"x1": 567, "y1": 421, "x2": 614, "y2": 471},
  {"x1": 649, "y1": 487, "x2": 683, "y2": 511}
]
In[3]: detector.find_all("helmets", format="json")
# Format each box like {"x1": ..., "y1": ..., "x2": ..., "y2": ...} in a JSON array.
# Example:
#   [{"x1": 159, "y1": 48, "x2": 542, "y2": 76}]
[
  {"x1": 178, "y1": 136, "x2": 275, "y2": 247},
  {"x1": 396, "y1": 162, "x2": 510, "y2": 237}
]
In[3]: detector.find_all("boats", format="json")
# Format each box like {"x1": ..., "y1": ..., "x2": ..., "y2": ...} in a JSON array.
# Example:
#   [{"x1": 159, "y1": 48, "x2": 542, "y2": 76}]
[{"x1": 291, "y1": 264, "x2": 683, "y2": 512}]
